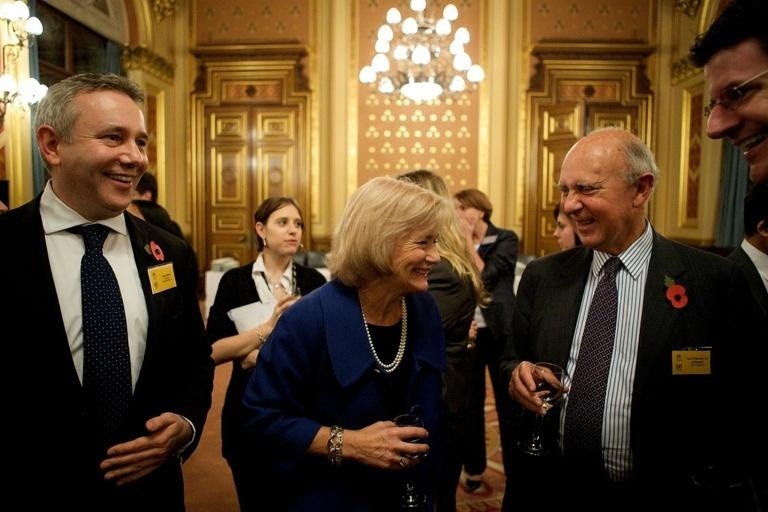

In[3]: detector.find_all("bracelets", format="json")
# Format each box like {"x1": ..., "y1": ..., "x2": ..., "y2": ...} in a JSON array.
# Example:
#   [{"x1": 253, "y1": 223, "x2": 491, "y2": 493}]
[
  {"x1": 255, "y1": 324, "x2": 267, "y2": 345},
  {"x1": 327, "y1": 425, "x2": 344, "y2": 465}
]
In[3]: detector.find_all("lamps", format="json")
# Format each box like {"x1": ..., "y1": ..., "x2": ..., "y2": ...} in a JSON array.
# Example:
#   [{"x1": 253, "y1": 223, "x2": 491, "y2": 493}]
[
  {"x1": 0, "y1": 73, "x2": 49, "y2": 114},
  {"x1": 359, "y1": 0, "x2": 484, "y2": 101},
  {"x1": 0, "y1": 0, "x2": 43, "y2": 69}
]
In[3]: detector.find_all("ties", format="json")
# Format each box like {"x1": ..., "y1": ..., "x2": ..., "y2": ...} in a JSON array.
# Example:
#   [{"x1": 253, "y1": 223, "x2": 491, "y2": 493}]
[
  {"x1": 66, "y1": 221, "x2": 134, "y2": 425},
  {"x1": 564, "y1": 256, "x2": 626, "y2": 488}
]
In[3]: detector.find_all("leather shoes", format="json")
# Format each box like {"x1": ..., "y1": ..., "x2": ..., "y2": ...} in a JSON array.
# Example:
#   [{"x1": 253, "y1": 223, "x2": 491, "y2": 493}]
[{"x1": 464, "y1": 480, "x2": 481, "y2": 493}]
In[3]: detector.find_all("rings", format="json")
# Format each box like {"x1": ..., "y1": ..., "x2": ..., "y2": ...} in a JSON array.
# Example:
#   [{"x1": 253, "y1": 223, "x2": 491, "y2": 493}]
[{"x1": 399, "y1": 454, "x2": 408, "y2": 466}]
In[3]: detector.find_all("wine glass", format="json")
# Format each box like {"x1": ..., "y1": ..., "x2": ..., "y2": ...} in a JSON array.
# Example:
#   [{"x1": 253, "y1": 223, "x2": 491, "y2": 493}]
[
  {"x1": 392, "y1": 415, "x2": 432, "y2": 509},
  {"x1": 513, "y1": 362, "x2": 565, "y2": 456},
  {"x1": 269, "y1": 273, "x2": 294, "y2": 302}
]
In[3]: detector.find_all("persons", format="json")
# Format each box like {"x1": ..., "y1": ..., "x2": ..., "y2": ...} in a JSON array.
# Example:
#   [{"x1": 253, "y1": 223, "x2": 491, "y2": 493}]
[
  {"x1": 689, "y1": 1, "x2": 768, "y2": 186},
  {"x1": 550, "y1": 199, "x2": 583, "y2": 252},
  {"x1": 497, "y1": 125, "x2": 767, "y2": 510},
  {"x1": 123, "y1": 200, "x2": 179, "y2": 234},
  {"x1": 724, "y1": 181, "x2": 768, "y2": 305},
  {"x1": 0, "y1": 70, "x2": 217, "y2": 510},
  {"x1": 207, "y1": 193, "x2": 328, "y2": 510},
  {"x1": 391, "y1": 170, "x2": 494, "y2": 493},
  {"x1": 445, "y1": 190, "x2": 519, "y2": 495},
  {"x1": 123, "y1": 171, "x2": 162, "y2": 204},
  {"x1": 239, "y1": 174, "x2": 456, "y2": 510}
]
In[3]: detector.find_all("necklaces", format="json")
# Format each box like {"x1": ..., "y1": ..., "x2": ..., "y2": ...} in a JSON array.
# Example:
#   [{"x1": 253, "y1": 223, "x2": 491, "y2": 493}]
[
  {"x1": 268, "y1": 278, "x2": 287, "y2": 292},
  {"x1": 358, "y1": 294, "x2": 408, "y2": 376}
]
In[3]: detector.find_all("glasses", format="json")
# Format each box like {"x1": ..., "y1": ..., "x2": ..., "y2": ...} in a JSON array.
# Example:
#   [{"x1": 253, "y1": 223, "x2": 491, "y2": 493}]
[{"x1": 704, "y1": 71, "x2": 768, "y2": 119}]
